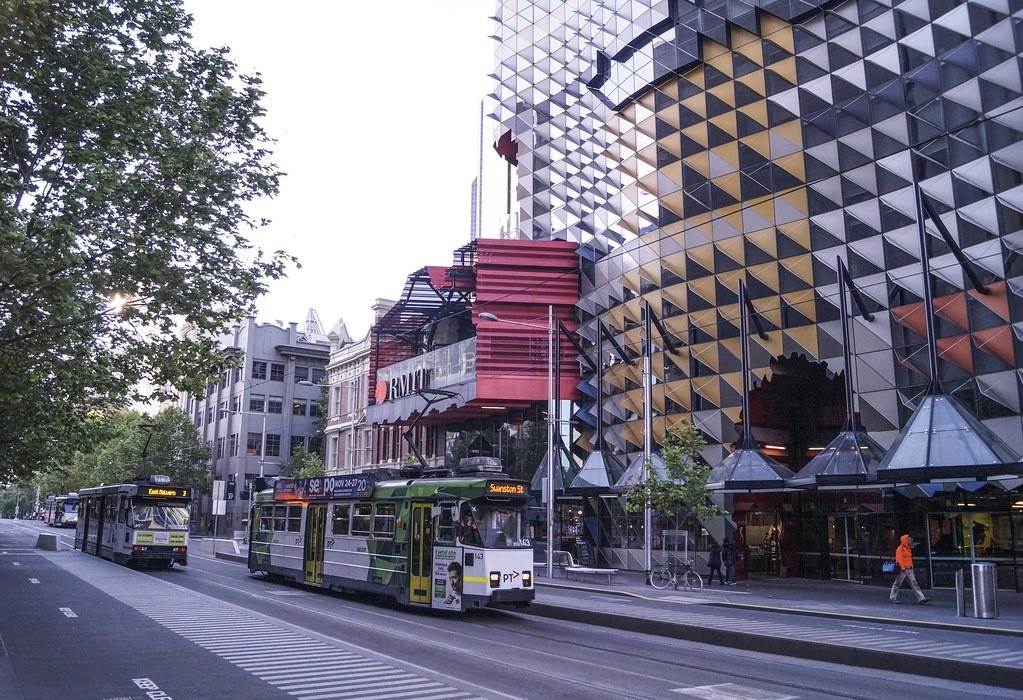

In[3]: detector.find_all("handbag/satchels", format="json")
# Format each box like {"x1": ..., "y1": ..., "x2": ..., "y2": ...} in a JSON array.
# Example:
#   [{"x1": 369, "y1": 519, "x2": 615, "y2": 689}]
[{"x1": 707, "y1": 561, "x2": 718, "y2": 569}]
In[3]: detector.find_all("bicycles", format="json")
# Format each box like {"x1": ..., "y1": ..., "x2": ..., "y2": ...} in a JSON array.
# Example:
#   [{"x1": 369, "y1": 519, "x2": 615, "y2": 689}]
[{"x1": 649, "y1": 555, "x2": 703, "y2": 592}]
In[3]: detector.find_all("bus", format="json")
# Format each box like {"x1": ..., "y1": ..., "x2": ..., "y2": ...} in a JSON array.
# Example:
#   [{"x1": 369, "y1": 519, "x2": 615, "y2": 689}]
[
  {"x1": 245, "y1": 388, "x2": 537, "y2": 617},
  {"x1": 70, "y1": 425, "x2": 194, "y2": 570},
  {"x1": 43, "y1": 479, "x2": 80, "y2": 528}
]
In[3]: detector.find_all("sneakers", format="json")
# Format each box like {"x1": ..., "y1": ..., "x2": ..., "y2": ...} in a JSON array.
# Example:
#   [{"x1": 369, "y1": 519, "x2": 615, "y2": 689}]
[
  {"x1": 724, "y1": 582, "x2": 728, "y2": 584},
  {"x1": 730, "y1": 582, "x2": 736, "y2": 585}
]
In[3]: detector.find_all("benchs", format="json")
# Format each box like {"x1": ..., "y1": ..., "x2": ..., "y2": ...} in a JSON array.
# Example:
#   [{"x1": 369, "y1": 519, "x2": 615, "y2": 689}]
[{"x1": 534, "y1": 550, "x2": 619, "y2": 585}]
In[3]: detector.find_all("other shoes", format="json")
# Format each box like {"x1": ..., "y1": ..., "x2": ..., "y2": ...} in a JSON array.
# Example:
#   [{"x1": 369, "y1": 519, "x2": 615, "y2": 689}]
[
  {"x1": 705, "y1": 581, "x2": 711, "y2": 584},
  {"x1": 720, "y1": 583, "x2": 724, "y2": 585},
  {"x1": 919, "y1": 596, "x2": 930, "y2": 604},
  {"x1": 888, "y1": 599, "x2": 899, "y2": 603}
]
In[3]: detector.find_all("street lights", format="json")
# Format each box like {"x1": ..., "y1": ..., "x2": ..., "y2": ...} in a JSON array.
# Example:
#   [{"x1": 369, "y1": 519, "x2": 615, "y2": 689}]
[
  {"x1": 298, "y1": 380, "x2": 356, "y2": 475},
  {"x1": 477, "y1": 302, "x2": 554, "y2": 580},
  {"x1": 302, "y1": 429, "x2": 322, "y2": 454},
  {"x1": 218, "y1": 401, "x2": 269, "y2": 478}
]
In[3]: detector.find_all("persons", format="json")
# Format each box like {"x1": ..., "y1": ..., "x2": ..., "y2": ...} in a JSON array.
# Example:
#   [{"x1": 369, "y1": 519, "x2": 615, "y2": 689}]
[
  {"x1": 460, "y1": 510, "x2": 477, "y2": 542},
  {"x1": 208, "y1": 523, "x2": 212, "y2": 537},
  {"x1": 888, "y1": 535, "x2": 930, "y2": 605},
  {"x1": 706, "y1": 539, "x2": 725, "y2": 586},
  {"x1": 33, "y1": 510, "x2": 36, "y2": 520},
  {"x1": 721, "y1": 537, "x2": 737, "y2": 585},
  {"x1": 443, "y1": 562, "x2": 463, "y2": 605}
]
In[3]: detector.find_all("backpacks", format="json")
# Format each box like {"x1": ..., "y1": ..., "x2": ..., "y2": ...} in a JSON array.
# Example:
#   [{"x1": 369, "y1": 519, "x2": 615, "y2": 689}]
[{"x1": 882, "y1": 554, "x2": 901, "y2": 575}]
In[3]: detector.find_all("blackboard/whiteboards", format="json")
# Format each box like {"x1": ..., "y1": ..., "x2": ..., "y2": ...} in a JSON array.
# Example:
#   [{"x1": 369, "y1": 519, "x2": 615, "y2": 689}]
[{"x1": 576, "y1": 541, "x2": 595, "y2": 566}]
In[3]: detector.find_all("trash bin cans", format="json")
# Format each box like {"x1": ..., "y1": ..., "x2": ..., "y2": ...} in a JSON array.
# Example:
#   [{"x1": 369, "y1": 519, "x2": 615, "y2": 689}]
[{"x1": 971, "y1": 562, "x2": 1000, "y2": 619}]
